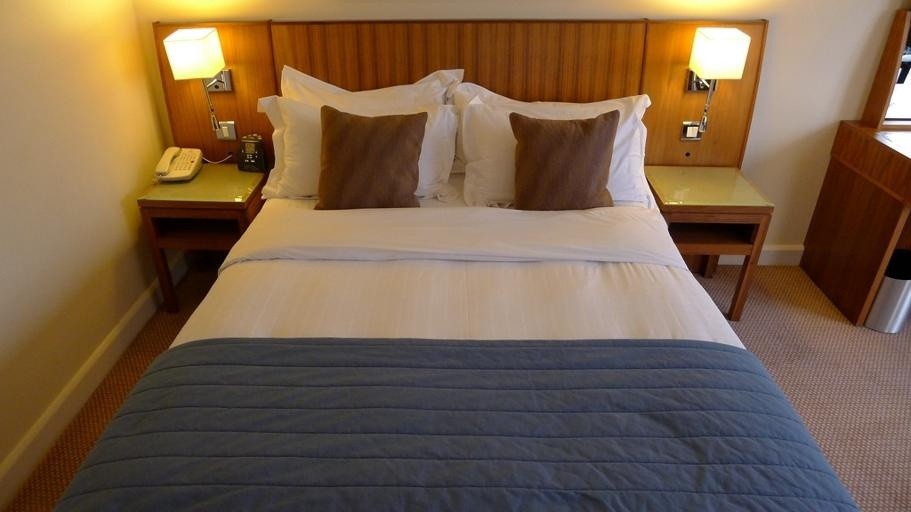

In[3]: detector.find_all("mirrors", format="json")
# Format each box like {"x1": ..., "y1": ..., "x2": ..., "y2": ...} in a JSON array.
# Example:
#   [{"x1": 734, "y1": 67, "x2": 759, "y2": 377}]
[{"x1": 884, "y1": 22, "x2": 910, "y2": 122}]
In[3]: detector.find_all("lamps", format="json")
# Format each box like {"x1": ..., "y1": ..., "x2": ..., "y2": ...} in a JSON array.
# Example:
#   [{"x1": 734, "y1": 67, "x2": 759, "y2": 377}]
[
  {"x1": 162, "y1": 28, "x2": 236, "y2": 142},
  {"x1": 684, "y1": 25, "x2": 752, "y2": 143}
]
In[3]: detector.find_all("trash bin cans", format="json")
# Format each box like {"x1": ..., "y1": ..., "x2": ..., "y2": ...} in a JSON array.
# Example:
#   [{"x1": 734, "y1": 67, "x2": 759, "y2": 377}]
[{"x1": 865, "y1": 249, "x2": 911, "y2": 335}]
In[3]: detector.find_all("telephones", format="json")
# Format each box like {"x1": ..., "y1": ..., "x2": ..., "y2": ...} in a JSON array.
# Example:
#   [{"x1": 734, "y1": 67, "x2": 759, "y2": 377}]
[{"x1": 154, "y1": 146, "x2": 203, "y2": 183}]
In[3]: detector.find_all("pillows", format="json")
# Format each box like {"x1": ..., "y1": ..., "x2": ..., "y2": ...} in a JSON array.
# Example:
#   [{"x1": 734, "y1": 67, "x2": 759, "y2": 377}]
[
  {"x1": 313, "y1": 102, "x2": 430, "y2": 208},
  {"x1": 459, "y1": 96, "x2": 647, "y2": 207},
  {"x1": 256, "y1": 91, "x2": 460, "y2": 203},
  {"x1": 451, "y1": 80, "x2": 650, "y2": 117},
  {"x1": 509, "y1": 110, "x2": 624, "y2": 208},
  {"x1": 274, "y1": 61, "x2": 464, "y2": 112}
]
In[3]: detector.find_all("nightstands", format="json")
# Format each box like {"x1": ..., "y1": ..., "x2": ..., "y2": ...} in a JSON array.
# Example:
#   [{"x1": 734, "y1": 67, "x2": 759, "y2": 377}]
[
  {"x1": 645, "y1": 161, "x2": 775, "y2": 326},
  {"x1": 139, "y1": 162, "x2": 266, "y2": 312}
]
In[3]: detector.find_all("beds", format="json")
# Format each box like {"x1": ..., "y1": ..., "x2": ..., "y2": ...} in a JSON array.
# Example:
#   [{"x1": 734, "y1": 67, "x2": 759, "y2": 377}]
[{"x1": 39, "y1": 19, "x2": 862, "y2": 512}]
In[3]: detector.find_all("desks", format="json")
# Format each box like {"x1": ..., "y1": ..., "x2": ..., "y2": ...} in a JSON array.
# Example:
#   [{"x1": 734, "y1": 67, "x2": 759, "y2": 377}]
[{"x1": 803, "y1": 1, "x2": 911, "y2": 327}]
